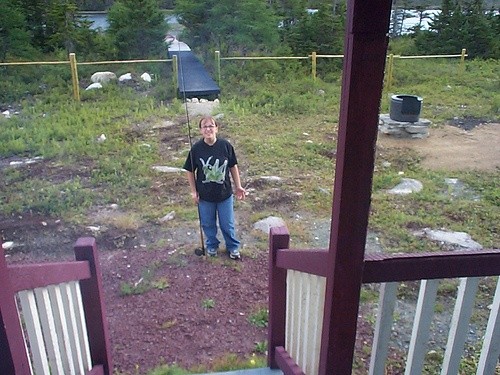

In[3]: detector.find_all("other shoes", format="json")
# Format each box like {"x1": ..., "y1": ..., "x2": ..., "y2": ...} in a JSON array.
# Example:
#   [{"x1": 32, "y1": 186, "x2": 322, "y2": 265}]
[
  {"x1": 230, "y1": 250, "x2": 240, "y2": 260},
  {"x1": 206, "y1": 248, "x2": 217, "y2": 256}
]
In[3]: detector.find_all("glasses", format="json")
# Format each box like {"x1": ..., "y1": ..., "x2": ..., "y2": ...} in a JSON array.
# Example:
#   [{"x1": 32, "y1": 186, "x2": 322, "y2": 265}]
[{"x1": 201, "y1": 125, "x2": 216, "y2": 129}]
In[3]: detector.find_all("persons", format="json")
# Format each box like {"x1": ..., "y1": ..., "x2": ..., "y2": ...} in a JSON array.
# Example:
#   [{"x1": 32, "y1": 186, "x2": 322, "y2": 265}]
[{"x1": 183, "y1": 116, "x2": 246, "y2": 259}]
